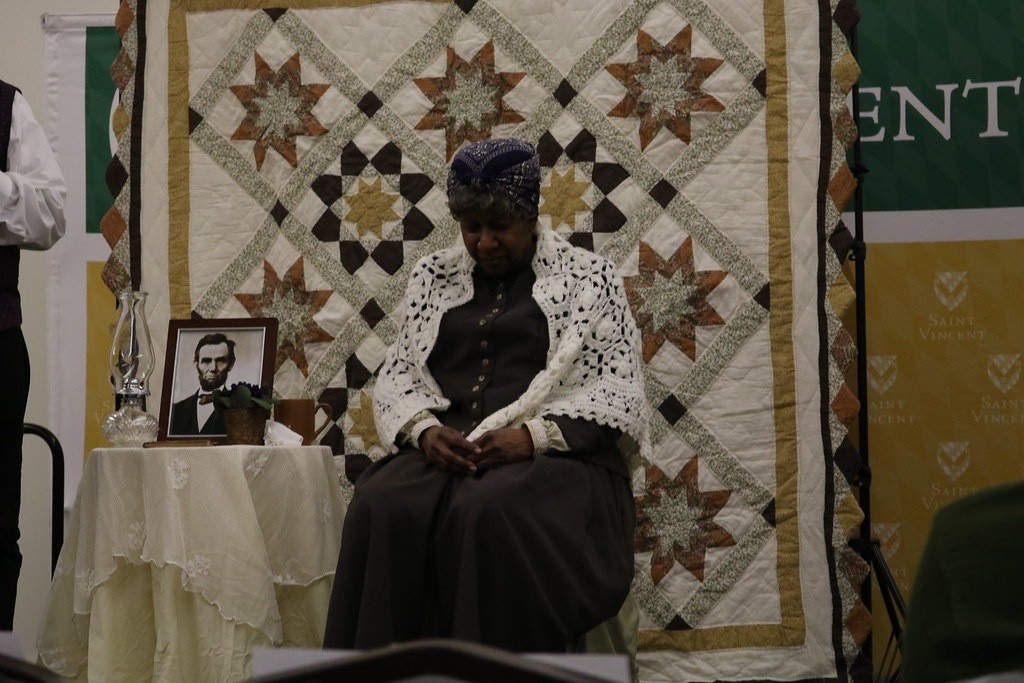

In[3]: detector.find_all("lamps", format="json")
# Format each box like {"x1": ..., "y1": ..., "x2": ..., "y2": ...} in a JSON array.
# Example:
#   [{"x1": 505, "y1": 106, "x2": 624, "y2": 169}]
[{"x1": 102, "y1": 289, "x2": 161, "y2": 447}]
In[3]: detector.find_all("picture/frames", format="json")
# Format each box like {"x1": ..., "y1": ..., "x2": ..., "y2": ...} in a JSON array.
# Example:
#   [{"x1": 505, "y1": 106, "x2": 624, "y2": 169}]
[{"x1": 162, "y1": 315, "x2": 280, "y2": 444}]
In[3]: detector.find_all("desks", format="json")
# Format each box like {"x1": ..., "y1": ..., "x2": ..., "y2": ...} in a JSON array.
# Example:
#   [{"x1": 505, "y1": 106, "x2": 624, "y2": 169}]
[{"x1": 32, "y1": 444, "x2": 349, "y2": 682}]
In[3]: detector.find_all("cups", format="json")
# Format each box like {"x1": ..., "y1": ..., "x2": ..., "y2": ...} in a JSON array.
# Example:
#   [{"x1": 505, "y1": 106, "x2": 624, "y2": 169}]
[{"x1": 274, "y1": 398, "x2": 332, "y2": 445}]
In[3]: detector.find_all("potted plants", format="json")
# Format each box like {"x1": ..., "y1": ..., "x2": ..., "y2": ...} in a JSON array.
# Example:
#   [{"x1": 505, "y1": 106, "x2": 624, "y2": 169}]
[{"x1": 215, "y1": 380, "x2": 281, "y2": 444}]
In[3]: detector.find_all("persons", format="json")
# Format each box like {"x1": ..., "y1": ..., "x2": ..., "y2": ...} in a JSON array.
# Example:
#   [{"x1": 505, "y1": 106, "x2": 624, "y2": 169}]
[
  {"x1": 0, "y1": 78, "x2": 68, "y2": 634},
  {"x1": 169, "y1": 333, "x2": 236, "y2": 435},
  {"x1": 320, "y1": 136, "x2": 654, "y2": 654}
]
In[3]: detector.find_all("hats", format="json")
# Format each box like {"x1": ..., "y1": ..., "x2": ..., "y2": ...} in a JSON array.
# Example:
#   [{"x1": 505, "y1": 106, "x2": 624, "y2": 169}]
[{"x1": 446, "y1": 139, "x2": 540, "y2": 218}]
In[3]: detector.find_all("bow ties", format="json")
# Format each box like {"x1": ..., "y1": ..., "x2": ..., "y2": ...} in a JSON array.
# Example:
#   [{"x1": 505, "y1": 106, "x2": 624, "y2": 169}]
[{"x1": 197, "y1": 394, "x2": 213, "y2": 405}]
